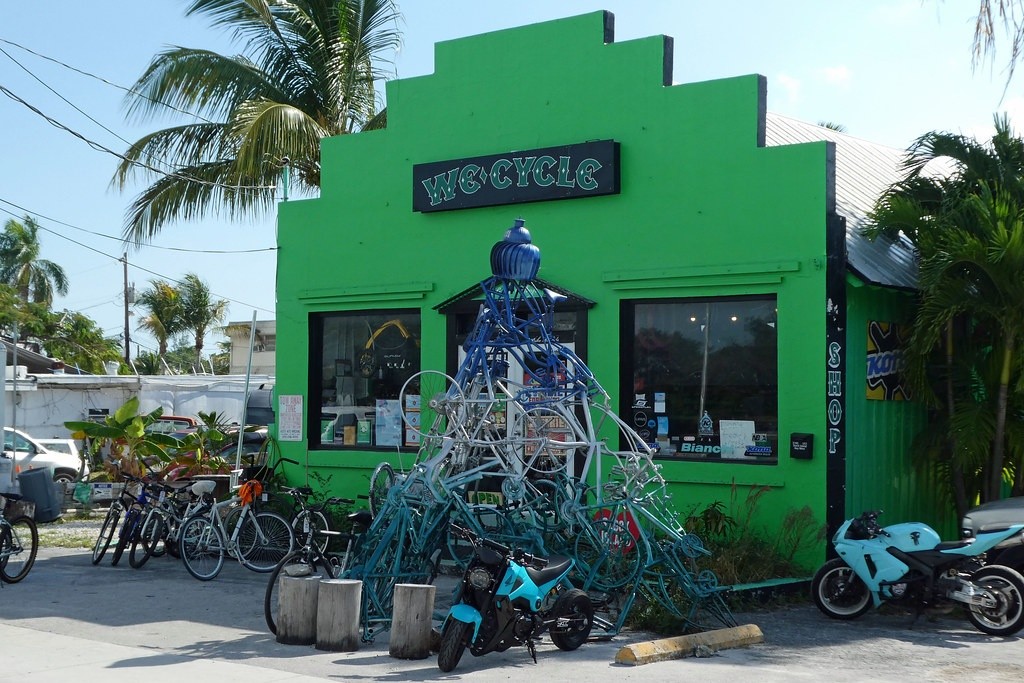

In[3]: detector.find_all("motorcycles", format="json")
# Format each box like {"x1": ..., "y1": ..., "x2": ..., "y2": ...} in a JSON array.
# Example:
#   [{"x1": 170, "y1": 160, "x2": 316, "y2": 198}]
[
  {"x1": 437, "y1": 523, "x2": 595, "y2": 672},
  {"x1": 811, "y1": 509, "x2": 1024, "y2": 638}
]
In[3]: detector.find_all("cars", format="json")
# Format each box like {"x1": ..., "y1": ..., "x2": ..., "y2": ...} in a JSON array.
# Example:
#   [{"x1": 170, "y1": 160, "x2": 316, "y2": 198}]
[
  {"x1": 141, "y1": 424, "x2": 269, "y2": 481},
  {"x1": 4, "y1": 426, "x2": 90, "y2": 484},
  {"x1": 4, "y1": 438, "x2": 89, "y2": 465},
  {"x1": 110, "y1": 415, "x2": 197, "y2": 458}
]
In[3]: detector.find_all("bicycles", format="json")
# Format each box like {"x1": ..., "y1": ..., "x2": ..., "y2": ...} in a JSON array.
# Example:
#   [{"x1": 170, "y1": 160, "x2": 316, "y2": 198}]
[
  {"x1": 336, "y1": 276, "x2": 740, "y2": 644},
  {"x1": 264, "y1": 485, "x2": 397, "y2": 635},
  {"x1": 93, "y1": 454, "x2": 333, "y2": 581},
  {"x1": 0, "y1": 492, "x2": 39, "y2": 584}
]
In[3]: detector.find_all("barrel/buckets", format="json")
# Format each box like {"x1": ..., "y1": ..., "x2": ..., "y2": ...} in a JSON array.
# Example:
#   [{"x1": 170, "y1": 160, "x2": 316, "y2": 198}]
[{"x1": 18, "y1": 466, "x2": 61, "y2": 523}]
